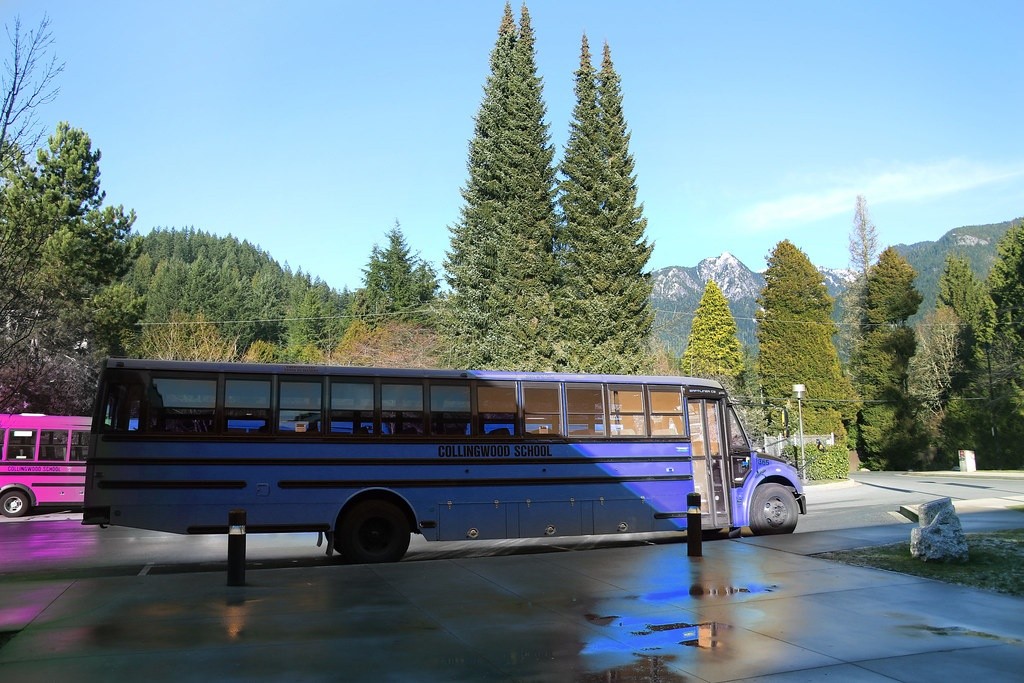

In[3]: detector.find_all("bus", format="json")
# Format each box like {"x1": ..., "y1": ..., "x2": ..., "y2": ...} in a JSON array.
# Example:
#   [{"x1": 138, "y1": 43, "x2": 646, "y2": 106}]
[
  {"x1": 81, "y1": 356, "x2": 806, "y2": 564},
  {"x1": 0, "y1": 413, "x2": 94, "y2": 519}
]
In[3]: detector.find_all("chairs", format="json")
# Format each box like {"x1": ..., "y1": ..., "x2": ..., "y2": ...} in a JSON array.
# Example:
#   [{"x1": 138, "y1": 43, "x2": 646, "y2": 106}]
[
  {"x1": 156, "y1": 409, "x2": 601, "y2": 434},
  {"x1": 10, "y1": 446, "x2": 86, "y2": 461}
]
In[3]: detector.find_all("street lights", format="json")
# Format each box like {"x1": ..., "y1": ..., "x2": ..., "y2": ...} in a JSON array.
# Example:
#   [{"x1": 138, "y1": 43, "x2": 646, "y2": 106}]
[{"x1": 792, "y1": 384, "x2": 807, "y2": 480}]
